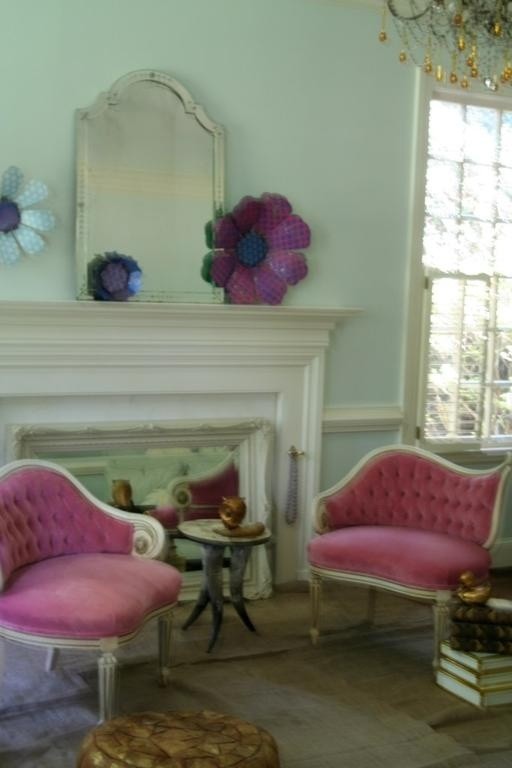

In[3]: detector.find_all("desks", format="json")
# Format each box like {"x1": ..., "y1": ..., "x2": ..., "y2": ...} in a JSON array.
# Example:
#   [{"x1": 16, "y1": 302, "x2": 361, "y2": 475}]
[{"x1": 177, "y1": 513, "x2": 269, "y2": 651}]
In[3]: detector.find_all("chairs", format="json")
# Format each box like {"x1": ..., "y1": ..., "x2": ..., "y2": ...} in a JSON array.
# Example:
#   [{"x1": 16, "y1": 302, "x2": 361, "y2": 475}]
[
  {"x1": 301, "y1": 435, "x2": 511, "y2": 675},
  {"x1": 139, "y1": 454, "x2": 240, "y2": 572},
  {"x1": 1, "y1": 456, "x2": 184, "y2": 726}
]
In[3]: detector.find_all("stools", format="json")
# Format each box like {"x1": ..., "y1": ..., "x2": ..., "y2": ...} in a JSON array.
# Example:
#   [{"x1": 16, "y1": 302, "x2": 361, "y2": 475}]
[{"x1": 76, "y1": 708, "x2": 279, "y2": 767}]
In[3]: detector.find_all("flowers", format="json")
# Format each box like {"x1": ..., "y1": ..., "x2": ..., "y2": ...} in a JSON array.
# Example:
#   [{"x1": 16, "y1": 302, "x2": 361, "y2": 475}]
[
  {"x1": 209, "y1": 190, "x2": 311, "y2": 307},
  {"x1": 88, "y1": 248, "x2": 142, "y2": 300},
  {"x1": 0, "y1": 164, "x2": 59, "y2": 262}
]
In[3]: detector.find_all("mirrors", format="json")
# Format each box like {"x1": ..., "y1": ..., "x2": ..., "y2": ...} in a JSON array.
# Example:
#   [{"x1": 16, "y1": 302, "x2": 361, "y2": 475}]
[
  {"x1": 12, "y1": 417, "x2": 277, "y2": 603},
  {"x1": 68, "y1": 62, "x2": 229, "y2": 304}
]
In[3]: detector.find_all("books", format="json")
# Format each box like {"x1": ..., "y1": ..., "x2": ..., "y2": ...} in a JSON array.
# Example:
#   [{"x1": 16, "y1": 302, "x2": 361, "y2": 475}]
[
  {"x1": 441, "y1": 656, "x2": 512, "y2": 690},
  {"x1": 439, "y1": 639, "x2": 512, "y2": 674},
  {"x1": 433, "y1": 671, "x2": 512, "y2": 710}
]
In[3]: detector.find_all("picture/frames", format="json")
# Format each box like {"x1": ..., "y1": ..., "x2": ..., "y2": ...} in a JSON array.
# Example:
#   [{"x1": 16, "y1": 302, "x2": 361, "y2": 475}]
[{"x1": 65, "y1": 463, "x2": 112, "y2": 501}]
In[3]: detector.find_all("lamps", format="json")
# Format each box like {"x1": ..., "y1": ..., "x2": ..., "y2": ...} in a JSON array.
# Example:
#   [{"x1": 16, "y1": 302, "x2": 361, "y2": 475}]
[{"x1": 379, "y1": 1, "x2": 511, "y2": 104}]
